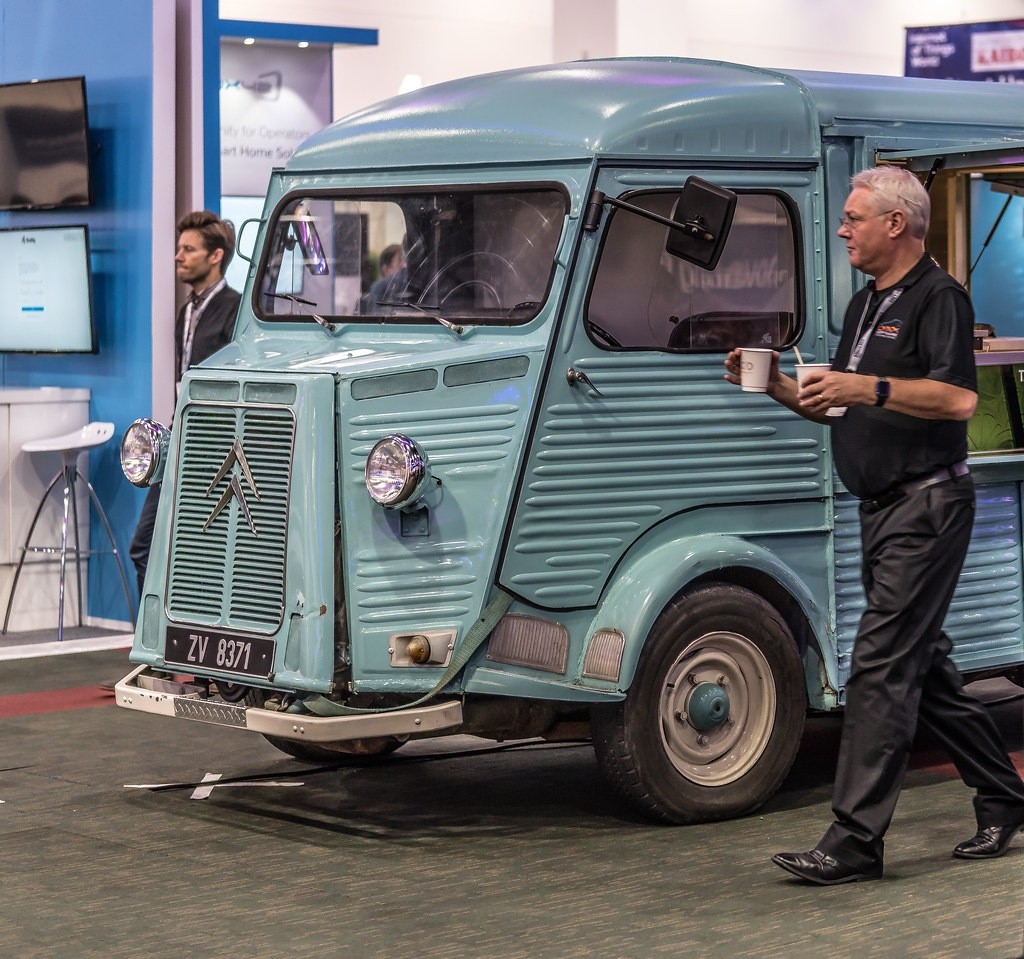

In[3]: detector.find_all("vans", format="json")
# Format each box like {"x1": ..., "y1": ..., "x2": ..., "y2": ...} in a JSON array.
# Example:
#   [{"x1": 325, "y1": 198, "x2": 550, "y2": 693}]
[{"x1": 109, "y1": 52, "x2": 1022, "y2": 832}]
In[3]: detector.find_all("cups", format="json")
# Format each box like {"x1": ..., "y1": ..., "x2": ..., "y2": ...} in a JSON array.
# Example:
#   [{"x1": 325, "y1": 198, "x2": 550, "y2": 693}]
[
  {"x1": 794, "y1": 363, "x2": 833, "y2": 408},
  {"x1": 740, "y1": 348, "x2": 773, "y2": 392}
]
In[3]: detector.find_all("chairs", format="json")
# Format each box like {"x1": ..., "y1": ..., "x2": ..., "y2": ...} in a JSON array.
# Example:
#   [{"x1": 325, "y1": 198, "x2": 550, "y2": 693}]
[{"x1": 667, "y1": 312, "x2": 795, "y2": 351}]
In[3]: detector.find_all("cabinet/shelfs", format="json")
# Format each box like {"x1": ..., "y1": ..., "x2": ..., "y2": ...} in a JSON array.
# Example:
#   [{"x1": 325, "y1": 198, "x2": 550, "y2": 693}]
[{"x1": 1, "y1": 387, "x2": 92, "y2": 632}]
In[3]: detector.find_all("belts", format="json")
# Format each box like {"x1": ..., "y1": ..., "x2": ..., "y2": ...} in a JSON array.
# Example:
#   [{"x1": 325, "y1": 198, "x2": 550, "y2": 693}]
[{"x1": 859, "y1": 460, "x2": 969, "y2": 516}]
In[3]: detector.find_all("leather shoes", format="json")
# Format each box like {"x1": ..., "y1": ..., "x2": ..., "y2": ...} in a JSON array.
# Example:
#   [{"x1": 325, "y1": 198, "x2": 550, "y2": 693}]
[
  {"x1": 951, "y1": 817, "x2": 1024, "y2": 858},
  {"x1": 772, "y1": 850, "x2": 874, "y2": 885}
]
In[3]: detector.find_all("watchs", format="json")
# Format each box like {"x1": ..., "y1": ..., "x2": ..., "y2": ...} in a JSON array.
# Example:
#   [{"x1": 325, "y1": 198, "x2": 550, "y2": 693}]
[{"x1": 874, "y1": 378, "x2": 891, "y2": 407}]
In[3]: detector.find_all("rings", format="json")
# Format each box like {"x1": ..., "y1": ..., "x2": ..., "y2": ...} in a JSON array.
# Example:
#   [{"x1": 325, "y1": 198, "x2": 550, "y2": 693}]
[{"x1": 819, "y1": 394, "x2": 823, "y2": 401}]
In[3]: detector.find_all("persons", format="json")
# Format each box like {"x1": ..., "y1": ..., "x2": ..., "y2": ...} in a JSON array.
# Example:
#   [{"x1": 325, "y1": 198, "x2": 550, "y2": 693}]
[
  {"x1": 363, "y1": 234, "x2": 408, "y2": 312},
  {"x1": 128, "y1": 210, "x2": 242, "y2": 599},
  {"x1": 723, "y1": 163, "x2": 1024, "y2": 886}
]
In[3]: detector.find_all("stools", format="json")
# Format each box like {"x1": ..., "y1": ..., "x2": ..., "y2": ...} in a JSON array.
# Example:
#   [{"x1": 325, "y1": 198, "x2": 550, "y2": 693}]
[{"x1": 1, "y1": 422, "x2": 137, "y2": 644}]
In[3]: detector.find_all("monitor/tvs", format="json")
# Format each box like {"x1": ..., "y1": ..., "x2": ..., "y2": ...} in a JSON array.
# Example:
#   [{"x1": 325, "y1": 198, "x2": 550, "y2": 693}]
[
  {"x1": 0, "y1": 75, "x2": 93, "y2": 211},
  {"x1": 0, "y1": 223, "x2": 99, "y2": 355}
]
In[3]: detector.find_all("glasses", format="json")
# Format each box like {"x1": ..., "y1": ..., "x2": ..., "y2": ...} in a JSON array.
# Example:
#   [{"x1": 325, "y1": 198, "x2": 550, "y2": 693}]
[{"x1": 838, "y1": 210, "x2": 894, "y2": 231}]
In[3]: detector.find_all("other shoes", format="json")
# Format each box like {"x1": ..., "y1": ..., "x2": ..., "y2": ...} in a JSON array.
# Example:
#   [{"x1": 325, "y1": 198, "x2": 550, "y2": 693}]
[{"x1": 98, "y1": 664, "x2": 172, "y2": 692}]
